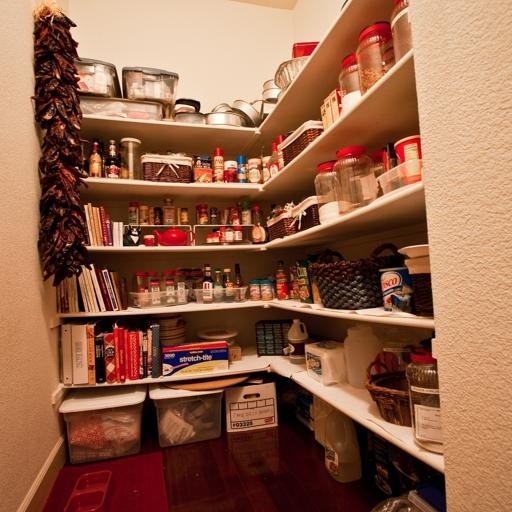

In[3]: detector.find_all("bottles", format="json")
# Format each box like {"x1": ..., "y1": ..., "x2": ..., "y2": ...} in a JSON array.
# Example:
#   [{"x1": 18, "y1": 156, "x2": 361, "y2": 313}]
[
  {"x1": 128, "y1": 197, "x2": 268, "y2": 244},
  {"x1": 343, "y1": 326, "x2": 382, "y2": 388},
  {"x1": 313, "y1": 145, "x2": 379, "y2": 224},
  {"x1": 131, "y1": 263, "x2": 244, "y2": 308},
  {"x1": 80, "y1": 137, "x2": 145, "y2": 179},
  {"x1": 248, "y1": 254, "x2": 321, "y2": 303},
  {"x1": 406, "y1": 353, "x2": 443, "y2": 456},
  {"x1": 338, "y1": 0, "x2": 413, "y2": 115},
  {"x1": 193, "y1": 136, "x2": 285, "y2": 185}
]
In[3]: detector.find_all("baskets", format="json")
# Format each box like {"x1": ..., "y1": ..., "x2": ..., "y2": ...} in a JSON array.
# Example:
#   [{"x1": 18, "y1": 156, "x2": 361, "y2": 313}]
[
  {"x1": 308, "y1": 244, "x2": 403, "y2": 309},
  {"x1": 277, "y1": 120, "x2": 322, "y2": 166},
  {"x1": 141, "y1": 155, "x2": 192, "y2": 183},
  {"x1": 295, "y1": 204, "x2": 320, "y2": 232},
  {"x1": 365, "y1": 361, "x2": 415, "y2": 426},
  {"x1": 266, "y1": 210, "x2": 296, "y2": 240}
]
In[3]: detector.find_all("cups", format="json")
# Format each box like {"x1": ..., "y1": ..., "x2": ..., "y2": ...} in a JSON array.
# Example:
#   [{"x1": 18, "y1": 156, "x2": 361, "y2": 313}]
[
  {"x1": 377, "y1": 268, "x2": 411, "y2": 311},
  {"x1": 394, "y1": 134, "x2": 422, "y2": 185}
]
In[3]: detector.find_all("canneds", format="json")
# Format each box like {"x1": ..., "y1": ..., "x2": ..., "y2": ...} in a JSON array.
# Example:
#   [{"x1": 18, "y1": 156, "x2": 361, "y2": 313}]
[
  {"x1": 128, "y1": 206, "x2": 139, "y2": 225},
  {"x1": 380, "y1": 268, "x2": 409, "y2": 311},
  {"x1": 197, "y1": 205, "x2": 208, "y2": 225},
  {"x1": 180, "y1": 208, "x2": 189, "y2": 225},
  {"x1": 154, "y1": 207, "x2": 162, "y2": 224},
  {"x1": 138, "y1": 205, "x2": 149, "y2": 224}
]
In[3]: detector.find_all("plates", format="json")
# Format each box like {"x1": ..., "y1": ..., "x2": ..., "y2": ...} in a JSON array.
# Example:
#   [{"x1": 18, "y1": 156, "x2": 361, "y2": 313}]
[{"x1": 397, "y1": 244, "x2": 430, "y2": 259}]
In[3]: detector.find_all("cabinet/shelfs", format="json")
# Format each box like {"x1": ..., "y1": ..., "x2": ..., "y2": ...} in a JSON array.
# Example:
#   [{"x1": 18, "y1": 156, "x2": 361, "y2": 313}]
[{"x1": 61, "y1": 0, "x2": 512, "y2": 512}]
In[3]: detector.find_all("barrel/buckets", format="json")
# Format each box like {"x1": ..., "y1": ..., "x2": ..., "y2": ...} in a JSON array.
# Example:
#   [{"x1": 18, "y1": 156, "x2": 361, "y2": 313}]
[{"x1": 288, "y1": 319, "x2": 309, "y2": 364}]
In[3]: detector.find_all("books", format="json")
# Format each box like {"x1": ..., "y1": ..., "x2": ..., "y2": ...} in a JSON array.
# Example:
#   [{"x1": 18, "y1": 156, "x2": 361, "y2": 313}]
[
  {"x1": 57, "y1": 321, "x2": 162, "y2": 384},
  {"x1": 81, "y1": 202, "x2": 113, "y2": 246},
  {"x1": 56, "y1": 263, "x2": 127, "y2": 312}
]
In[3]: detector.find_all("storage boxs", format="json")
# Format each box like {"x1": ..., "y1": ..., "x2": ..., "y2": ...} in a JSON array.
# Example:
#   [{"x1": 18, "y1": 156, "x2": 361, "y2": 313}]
[
  {"x1": 121, "y1": 66, "x2": 179, "y2": 105},
  {"x1": 58, "y1": 385, "x2": 147, "y2": 465},
  {"x1": 162, "y1": 340, "x2": 229, "y2": 378},
  {"x1": 72, "y1": 56, "x2": 123, "y2": 97},
  {"x1": 74, "y1": 95, "x2": 163, "y2": 121},
  {"x1": 148, "y1": 383, "x2": 227, "y2": 448},
  {"x1": 226, "y1": 428, "x2": 282, "y2": 481},
  {"x1": 225, "y1": 379, "x2": 278, "y2": 433}
]
